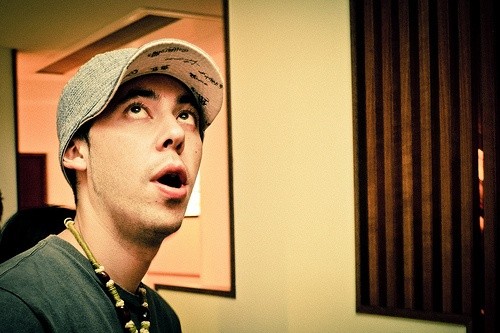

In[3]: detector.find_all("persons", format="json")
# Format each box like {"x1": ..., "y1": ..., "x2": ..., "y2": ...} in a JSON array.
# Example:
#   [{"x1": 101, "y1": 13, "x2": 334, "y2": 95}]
[{"x1": 2, "y1": 34, "x2": 226, "y2": 333}]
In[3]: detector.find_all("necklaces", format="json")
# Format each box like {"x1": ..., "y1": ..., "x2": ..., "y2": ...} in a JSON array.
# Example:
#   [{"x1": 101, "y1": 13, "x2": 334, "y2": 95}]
[{"x1": 62, "y1": 216, "x2": 158, "y2": 333}]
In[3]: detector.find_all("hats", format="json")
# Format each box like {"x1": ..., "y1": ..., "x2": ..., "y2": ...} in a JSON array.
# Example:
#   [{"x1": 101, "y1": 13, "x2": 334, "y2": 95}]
[{"x1": 55, "y1": 38, "x2": 222, "y2": 188}]
